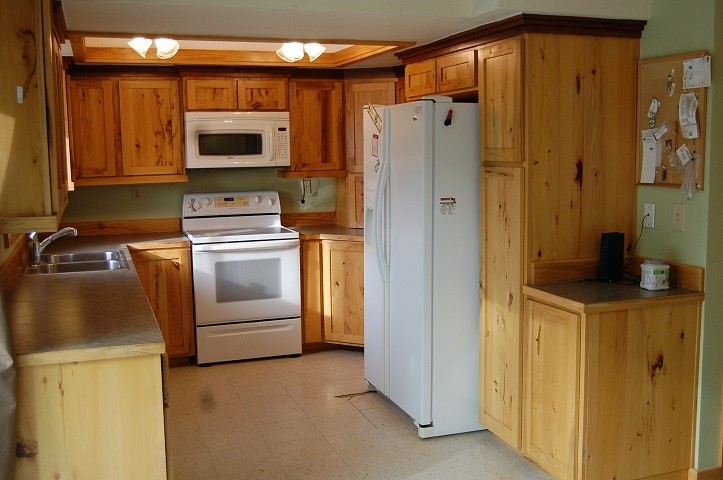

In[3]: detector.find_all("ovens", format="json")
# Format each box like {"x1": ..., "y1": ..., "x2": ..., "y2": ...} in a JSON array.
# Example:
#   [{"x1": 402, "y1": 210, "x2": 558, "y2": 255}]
[{"x1": 191, "y1": 240, "x2": 302, "y2": 364}]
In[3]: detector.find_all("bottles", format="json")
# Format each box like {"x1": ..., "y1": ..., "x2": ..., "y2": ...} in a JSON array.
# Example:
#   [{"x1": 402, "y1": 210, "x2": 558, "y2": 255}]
[{"x1": 639, "y1": 258, "x2": 670, "y2": 290}]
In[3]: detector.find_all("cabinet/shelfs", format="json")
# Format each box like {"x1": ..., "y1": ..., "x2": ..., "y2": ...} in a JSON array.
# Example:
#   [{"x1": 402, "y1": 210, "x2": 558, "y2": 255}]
[
  {"x1": 480, "y1": 166, "x2": 526, "y2": 447},
  {"x1": 525, "y1": 302, "x2": 582, "y2": 479},
  {"x1": 119, "y1": 80, "x2": 182, "y2": 174},
  {"x1": 42, "y1": 0, "x2": 67, "y2": 211},
  {"x1": 290, "y1": 77, "x2": 344, "y2": 170},
  {"x1": 131, "y1": 248, "x2": 191, "y2": 356},
  {"x1": 69, "y1": 80, "x2": 116, "y2": 176},
  {"x1": 405, "y1": 49, "x2": 478, "y2": 98},
  {"x1": 187, "y1": 79, "x2": 286, "y2": 109},
  {"x1": 321, "y1": 239, "x2": 364, "y2": 344},
  {"x1": 345, "y1": 80, "x2": 395, "y2": 172},
  {"x1": 478, "y1": 37, "x2": 525, "y2": 161}
]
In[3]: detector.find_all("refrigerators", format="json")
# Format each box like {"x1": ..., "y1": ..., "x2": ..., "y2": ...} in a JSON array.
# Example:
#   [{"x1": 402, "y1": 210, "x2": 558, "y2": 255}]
[{"x1": 362, "y1": 95, "x2": 488, "y2": 438}]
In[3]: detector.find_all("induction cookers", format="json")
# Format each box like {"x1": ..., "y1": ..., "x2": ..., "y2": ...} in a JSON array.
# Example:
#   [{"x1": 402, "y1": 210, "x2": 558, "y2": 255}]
[{"x1": 181, "y1": 191, "x2": 299, "y2": 245}]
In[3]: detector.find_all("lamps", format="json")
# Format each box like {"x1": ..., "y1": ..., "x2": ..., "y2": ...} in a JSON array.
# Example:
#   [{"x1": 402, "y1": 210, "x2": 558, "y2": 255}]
[
  {"x1": 276, "y1": 43, "x2": 326, "y2": 62},
  {"x1": 127, "y1": 38, "x2": 179, "y2": 58}
]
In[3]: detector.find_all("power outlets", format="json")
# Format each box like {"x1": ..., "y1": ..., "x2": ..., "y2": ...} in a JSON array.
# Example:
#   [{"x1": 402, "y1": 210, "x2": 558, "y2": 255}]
[
  {"x1": 301, "y1": 179, "x2": 311, "y2": 195},
  {"x1": 671, "y1": 203, "x2": 686, "y2": 232},
  {"x1": 644, "y1": 203, "x2": 655, "y2": 229}
]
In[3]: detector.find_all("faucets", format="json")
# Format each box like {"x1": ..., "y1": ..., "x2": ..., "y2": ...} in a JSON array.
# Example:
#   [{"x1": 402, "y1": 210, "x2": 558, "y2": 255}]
[{"x1": 31, "y1": 227, "x2": 77, "y2": 267}]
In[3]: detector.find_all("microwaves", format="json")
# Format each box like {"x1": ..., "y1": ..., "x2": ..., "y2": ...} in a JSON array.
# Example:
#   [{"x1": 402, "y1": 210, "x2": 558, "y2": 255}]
[{"x1": 183, "y1": 111, "x2": 291, "y2": 168}]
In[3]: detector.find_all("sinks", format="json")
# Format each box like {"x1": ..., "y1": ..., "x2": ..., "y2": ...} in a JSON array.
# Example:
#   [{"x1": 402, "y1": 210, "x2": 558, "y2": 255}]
[
  {"x1": 34, "y1": 261, "x2": 120, "y2": 275},
  {"x1": 40, "y1": 252, "x2": 118, "y2": 263}
]
